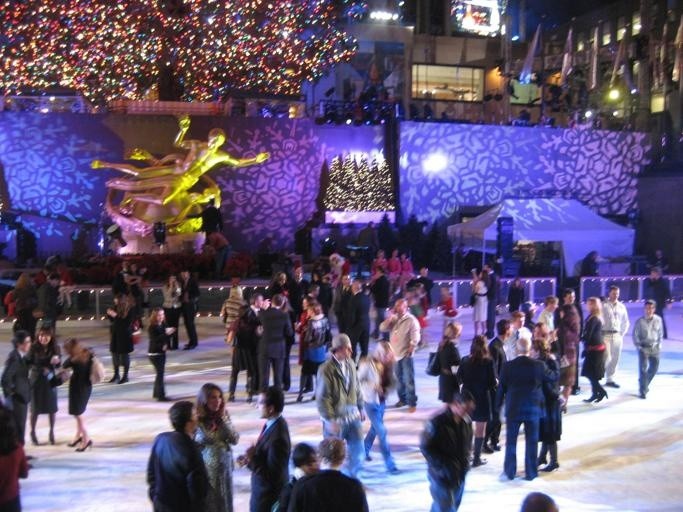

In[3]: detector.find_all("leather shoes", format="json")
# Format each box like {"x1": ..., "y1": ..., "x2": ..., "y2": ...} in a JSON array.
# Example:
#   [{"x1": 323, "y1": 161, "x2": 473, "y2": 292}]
[
  {"x1": 607, "y1": 382, "x2": 620, "y2": 388},
  {"x1": 493, "y1": 444, "x2": 501, "y2": 451},
  {"x1": 483, "y1": 445, "x2": 493, "y2": 453},
  {"x1": 641, "y1": 393, "x2": 646, "y2": 398},
  {"x1": 184, "y1": 345, "x2": 195, "y2": 351},
  {"x1": 157, "y1": 396, "x2": 169, "y2": 401}
]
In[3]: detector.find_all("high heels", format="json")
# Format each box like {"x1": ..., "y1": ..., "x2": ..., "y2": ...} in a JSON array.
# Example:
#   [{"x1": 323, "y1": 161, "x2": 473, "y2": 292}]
[
  {"x1": 541, "y1": 463, "x2": 561, "y2": 473},
  {"x1": 295, "y1": 396, "x2": 304, "y2": 405},
  {"x1": 30, "y1": 431, "x2": 40, "y2": 445},
  {"x1": 229, "y1": 394, "x2": 235, "y2": 404},
  {"x1": 596, "y1": 391, "x2": 608, "y2": 402},
  {"x1": 583, "y1": 394, "x2": 598, "y2": 402},
  {"x1": 246, "y1": 398, "x2": 252, "y2": 404},
  {"x1": 75, "y1": 440, "x2": 92, "y2": 452},
  {"x1": 536, "y1": 457, "x2": 548, "y2": 466},
  {"x1": 68, "y1": 437, "x2": 82, "y2": 447},
  {"x1": 49, "y1": 434, "x2": 55, "y2": 445}
]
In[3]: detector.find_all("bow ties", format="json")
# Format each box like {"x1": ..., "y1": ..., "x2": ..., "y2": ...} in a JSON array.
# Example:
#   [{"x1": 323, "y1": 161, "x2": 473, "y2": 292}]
[{"x1": 122, "y1": 270, "x2": 130, "y2": 276}]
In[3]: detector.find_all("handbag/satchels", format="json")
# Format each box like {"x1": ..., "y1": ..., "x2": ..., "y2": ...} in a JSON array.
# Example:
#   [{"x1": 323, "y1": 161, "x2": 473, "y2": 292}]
[
  {"x1": 424, "y1": 350, "x2": 444, "y2": 376},
  {"x1": 49, "y1": 362, "x2": 68, "y2": 386},
  {"x1": 469, "y1": 284, "x2": 477, "y2": 305},
  {"x1": 88, "y1": 352, "x2": 107, "y2": 386}
]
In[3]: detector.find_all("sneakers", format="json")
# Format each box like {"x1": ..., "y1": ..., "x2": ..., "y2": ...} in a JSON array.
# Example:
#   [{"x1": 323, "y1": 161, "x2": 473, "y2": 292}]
[
  {"x1": 390, "y1": 468, "x2": 401, "y2": 474},
  {"x1": 366, "y1": 456, "x2": 371, "y2": 461},
  {"x1": 396, "y1": 401, "x2": 406, "y2": 407},
  {"x1": 408, "y1": 407, "x2": 417, "y2": 413}
]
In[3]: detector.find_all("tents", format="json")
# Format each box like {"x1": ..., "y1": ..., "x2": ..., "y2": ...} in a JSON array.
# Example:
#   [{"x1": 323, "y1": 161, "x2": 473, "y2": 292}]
[{"x1": 446, "y1": 198, "x2": 635, "y2": 275}]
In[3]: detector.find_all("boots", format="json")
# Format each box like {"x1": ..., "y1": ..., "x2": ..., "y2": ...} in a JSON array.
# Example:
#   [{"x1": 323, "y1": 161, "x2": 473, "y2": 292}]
[
  {"x1": 473, "y1": 437, "x2": 488, "y2": 466},
  {"x1": 109, "y1": 359, "x2": 120, "y2": 383},
  {"x1": 118, "y1": 356, "x2": 129, "y2": 384}
]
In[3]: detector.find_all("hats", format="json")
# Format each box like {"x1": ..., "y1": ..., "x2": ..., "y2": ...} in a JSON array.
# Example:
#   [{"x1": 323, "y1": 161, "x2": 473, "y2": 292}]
[{"x1": 330, "y1": 332, "x2": 348, "y2": 353}]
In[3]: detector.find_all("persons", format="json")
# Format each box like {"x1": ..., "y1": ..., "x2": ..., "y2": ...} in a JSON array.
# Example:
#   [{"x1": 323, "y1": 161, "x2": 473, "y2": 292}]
[
  {"x1": 532, "y1": 339, "x2": 562, "y2": 472},
  {"x1": 32, "y1": 269, "x2": 48, "y2": 289},
  {"x1": 580, "y1": 251, "x2": 598, "y2": 275},
  {"x1": 227, "y1": 297, "x2": 272, "y2": 403},
  {"x1": 63, "y1": 337, "x2": 94, "y2": 452},
  {"x1": 45, "y1": 256, "x2": 63, "y2": 282},
  {"x1": 88, "y1": 251, "x2": 101, "y2": 263},
  {"x1": 553, "y1": 288, "x2": 584, "y2": 395},
  {"x1": 357, "y1": 340, "x2": 403, "y2": 475},
  {"x1": 558, "y1": 304, "x2": 581, "y2": 414},
  {"x1": 146, "y1": 400, "x2": 206, "y2": 512},
  {"x1": 279, "y1": 443, "x2": 319, "y2": 512},
  {"x1": 435, "y1": 285, "x2": 463, "y2": 334},
  {"x1": 219, "y1": 284, "x2": 247, "y2": 355},
  {"x1": 494, "y1": 311, "x2": 532, "y2": 413},
  {"x1": 347, "y1": 278, "x2": 369, "y2": 369},
  {"x1": 414, "y1": 265, "x2": 434, "y2": 309},
  {"x1": 310, "y1": 270, "x2": 326, "y2": 315},
  {"x1": 456, "y1": 334, "x2": 497, "y2": 467},
  {"x1": 298, "y1": 301, "x2": 300, "y2": 302},
  {"x1": 236, "y1": 292, "x2": 264, "y2": 396},
  {"x1": 531, "y1": 322, "x2": 550, "y2": 343},
  {"x1": 520, "y1": 302, "x2": 538, "y2": 331},
  {"x1": 256, "y1": 232, "x2": 274, "y2": 255},
  {"x1": 519, "y1": 491, "x2": 565, "y2": 512},
  {"x1": 482, "y1": 263, "x2": 497, "y2": 339},
  {"x1": 228, "y1": 276, "x2": 243, "y2": 300},
  {"x1": 37, "y1": 272, "x2": 60, "y2": 342},
  {"x1": 256, "y1": 294, "x2": 293, "y2": 393},
  {"x1": 357, "y1": 221, "x2": 380, "y2": 249},
  {"x1": 129, "y1": 263, "x2": 145, "y2": 329},
  {"x1": 321, "y1": 253, "x2": 351, "y2": 285},
  {"x1": 0, "y1": 329, "x2": 33, "y2": 461},
  {"x1": 179, "y1": 268, "x2": 201, "y2": 350},
  {"x1": 197, "y1": 198, "x2": 224, "y2": 233},
  {"x1": 481, "y1": 319, "x2": 514, "y2": 454},
  {"x1": 438, "y1": 321, "x2": 464, "y2": 419},
  {"x1": 267, "y1": 271, "x2": 299, "y2": 330},
  {"x1": 89, "y1": 114, "x2": 270, "y2": 224},
  {"x1": 580, "y1": 296, "x2": 608, "y2": 403},
  {"x1": 332, "y1": 260, "x2": 350, "y2": 291},
  {"x1": 473, "y1": 270, "x2": 488, "y2": 341},
  {"x1": 369, "y1": 265, "x2": 391, "y2": 340},
  {"x1": 221, "y1": 249, "x2": 255, "y2": 279},
  {"x1": 420, "y1": 389, "x2": 477, "y2": 512},
  {"x1": 399, "y1": 250, "x2": 413, "y2": 274},
  {"x1": 387, "y1": 248, "x2": 402, "y2": 279},
  {"x1": 495, "y1": 337, "x2": 560, "y2": 482},
  {"x1": 288, "y1": 437, "x2": 369, "y2": 512},
  {"x1": 112, "y1": 260, "x2": 147, "y2": 294},
  {"x1": 538, "y1": 296, "x2": 561, "y2": 356},
  {"x1": 147, "y1": 306, "x2": 176, "y2": 402},
  {"x1": 237, "y1": 386, "x2": 291, "y2": 512},
  {"x1": 370, "y1": 249, "x2": 388, "y2": 281},
  {"x1": 296, "y1": 303, "x2": 330, "y2": 404},
  {"x1": 5, "y1": 288, "x2": 19, "y2": 322},
  {"x1": 601, "y1": 285, "x2": 630, "y2": 388},
  {"x1": 13, "y1": 272, "x2": 38, "y2": 336},
  {"x1": 294, "y1": 296, "x2": 318, "y2": 393},
  {"x1": 31, "y1": 327, "x2": 61, "y2": 445},
  {"x1": 632, "y1": 299, "x2": 664, "y2": 399},
  {"x1": 643, "y1": 266, "x2": 673, "y2": 340},
  {"x1": 196, "y1": 382, "x2": 240, "y2": 512},
  {"x1": 160, "y1": 273, "x2": 184, "y2": 350},
  {"x1": 315, "y1": 334, "x2": 367, "y2": 481},
  {"x1": 645, "y1": 249, "x2": 669, "y2": 276},
  {"x1": 506, "y1": 277, "x2": 525, "y2": 313},
  {"x1": 105, "y1": 290, "x2": 137, "y2": 384},
  {"x1": 290, "y1": 259, "x2": 310, "y2": 290},
  {"x1": 0, "y1": 393, "x2": 28, "y2": 512},
  {"x1": 207, "y1": 230, "x2": 232, "y2": 282},
  {"x1": 311, "y1": 262, "x2": 323, "y2": 273},
  {"x1": 298, "y1": 284, "x2": 328, "y2": 322},
  {"x1": 378, "y1": 298, "x2": 422, "y2": 414},
  {"x1": 338, "y1": 274, "x2": 353, "y2": 333}
]
